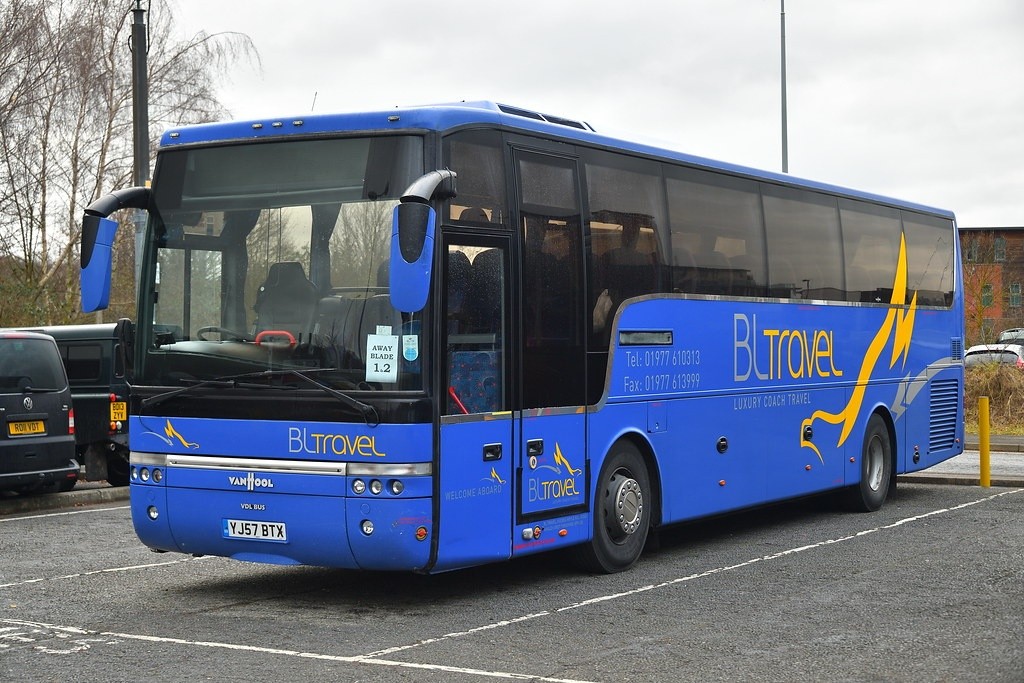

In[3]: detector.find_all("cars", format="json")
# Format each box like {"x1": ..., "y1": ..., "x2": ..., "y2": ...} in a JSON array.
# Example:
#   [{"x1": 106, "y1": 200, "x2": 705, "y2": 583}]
[{"x1": 964, "y1": 328, "x2": 1024, "y2": 374}]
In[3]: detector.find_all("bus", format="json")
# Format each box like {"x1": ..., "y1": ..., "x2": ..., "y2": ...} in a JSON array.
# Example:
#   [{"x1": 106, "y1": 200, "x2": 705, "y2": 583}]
[{"x1": 81, "y1": 98, "x2": 963, "y2": 575}]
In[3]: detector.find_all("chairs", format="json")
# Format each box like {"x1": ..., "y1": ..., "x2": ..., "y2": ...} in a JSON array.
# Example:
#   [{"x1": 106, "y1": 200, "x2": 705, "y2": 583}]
[
  {"x1": 254, "y1": 262, "x2": 321, "y2": 342},
  {"x1": 650, "y1": 246, "x2": 702, "y2": 293},
  {"x1": 598, "y1": 248, "x2": 650, "y2": 299},
  {"x1": 376, "y1": 249, "x2": 501, "y2": 330},
  {"x1": 771, "y1": 261, "x2": 895, "y2": 303},
  {"x1": 730, "y1": 255, "x2": 758, "y2": 296},
  {"x1": 695, "y1": 251, "x2": 733, "y2": 295}
]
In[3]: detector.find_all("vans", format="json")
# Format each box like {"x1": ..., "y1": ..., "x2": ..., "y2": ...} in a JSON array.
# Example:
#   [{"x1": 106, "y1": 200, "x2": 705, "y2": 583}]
[{"x1": 0, "y1": 322, "x2": 185, "y2": 498}]
